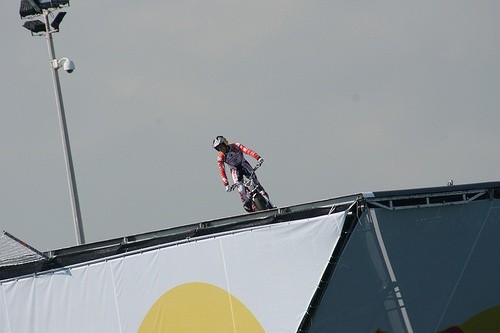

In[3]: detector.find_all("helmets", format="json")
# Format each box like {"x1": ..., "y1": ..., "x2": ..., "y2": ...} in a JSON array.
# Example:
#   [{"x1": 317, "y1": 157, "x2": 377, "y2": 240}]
[{"x1": 213, "y1": 136, "x2": 229, "y2": 152}]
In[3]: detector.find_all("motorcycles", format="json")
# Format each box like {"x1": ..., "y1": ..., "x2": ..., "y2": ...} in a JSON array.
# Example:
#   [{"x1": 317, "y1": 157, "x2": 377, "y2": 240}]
[{"x1": 228, "y1": 159, "x2": 268, "y2": 212}]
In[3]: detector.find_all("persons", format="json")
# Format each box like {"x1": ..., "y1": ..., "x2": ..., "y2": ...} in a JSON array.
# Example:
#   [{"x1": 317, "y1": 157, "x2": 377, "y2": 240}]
[{"x1": 213, "y1": 135, "x2": 273, "y2": 212}]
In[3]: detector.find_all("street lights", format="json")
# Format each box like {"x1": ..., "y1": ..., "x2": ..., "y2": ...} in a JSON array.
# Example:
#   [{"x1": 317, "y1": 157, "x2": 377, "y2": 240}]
[{"x1": 17, "y1": 0, "x2": 87, "y2": 246}]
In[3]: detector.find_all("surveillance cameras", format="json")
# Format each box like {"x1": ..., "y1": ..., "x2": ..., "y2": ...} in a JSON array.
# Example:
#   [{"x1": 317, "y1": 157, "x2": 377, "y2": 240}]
[{"x1": 63, "y1": 60, "x2": 75, "y2": 73}]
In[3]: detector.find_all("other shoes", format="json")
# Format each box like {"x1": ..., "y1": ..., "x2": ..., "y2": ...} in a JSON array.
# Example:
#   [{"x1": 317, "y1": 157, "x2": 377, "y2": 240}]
[
  {"x1": 244, "y1": 203, "x2": 254, "y2": 212},
  {"x1": 267, "y1": 202, "x2": 274, "y2": 209}
]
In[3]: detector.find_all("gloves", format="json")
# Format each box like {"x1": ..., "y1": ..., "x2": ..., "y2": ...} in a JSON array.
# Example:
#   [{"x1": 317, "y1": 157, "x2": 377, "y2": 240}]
[
  {"x1": 258, "y1": 158, "x2": 264, "y2": 165},
  {"x1": 225, "y1": 185, "x2": 231, "y2": 192}
]
